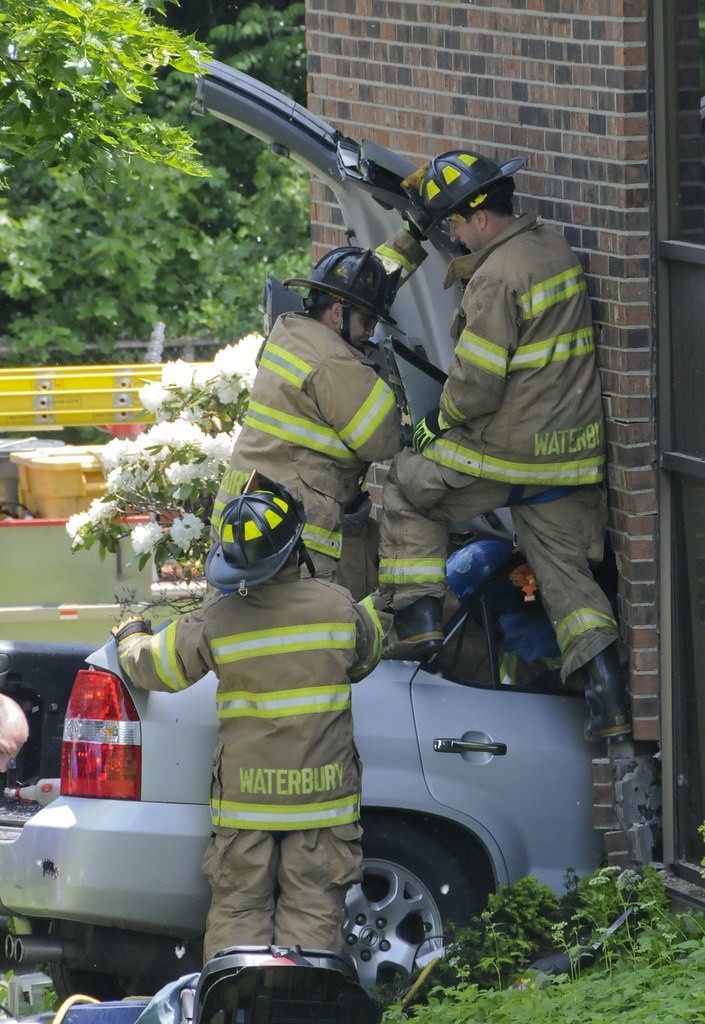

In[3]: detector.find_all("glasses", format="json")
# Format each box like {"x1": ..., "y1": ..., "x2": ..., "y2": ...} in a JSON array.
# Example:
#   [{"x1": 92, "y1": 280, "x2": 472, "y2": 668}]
[{"x1": 436, "y1": 209, "x2": 474, "y2": 235}]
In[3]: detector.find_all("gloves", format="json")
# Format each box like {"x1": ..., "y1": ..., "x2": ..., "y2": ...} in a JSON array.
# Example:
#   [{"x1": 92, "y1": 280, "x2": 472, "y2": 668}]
[
  {"x1": 110, "y1": 614, "x2": 154, "y2": 648},
  {"x1": 412, "y1": 406, "x2": 454, "y2": 453}
]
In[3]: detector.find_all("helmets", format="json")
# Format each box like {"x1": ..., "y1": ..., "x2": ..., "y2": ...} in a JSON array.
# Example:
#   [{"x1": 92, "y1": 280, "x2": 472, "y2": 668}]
[
  {"x1": 282, "y1": 247, "x2": 401, "y2": 325},
  {"x1": 203, "y1": 491, "x2": 307, "y2": 589},
  {"x1": 419, "y1": 150, "x2": 525, "y2": 236}
]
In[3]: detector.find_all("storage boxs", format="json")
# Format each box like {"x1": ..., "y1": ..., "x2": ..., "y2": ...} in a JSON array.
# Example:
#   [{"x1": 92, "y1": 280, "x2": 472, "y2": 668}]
[{"x1": 8, "y1": 444, "x2": 111, "y2": 520}]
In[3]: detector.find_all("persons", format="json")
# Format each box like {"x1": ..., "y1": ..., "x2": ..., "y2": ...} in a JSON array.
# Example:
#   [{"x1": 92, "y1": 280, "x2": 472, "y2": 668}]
[
  {"x1": 208, "y1": 217, "x2": 428, "y2": 599},
  {"x1": 108, "y1": 488, "x2": 384, "y2": 965},
  {"x1": 0, "y1": 694, "x2": 31, "y2": 774},
  {"x1": 363, "y1": 151, "x2": 633, "y2": 740}
]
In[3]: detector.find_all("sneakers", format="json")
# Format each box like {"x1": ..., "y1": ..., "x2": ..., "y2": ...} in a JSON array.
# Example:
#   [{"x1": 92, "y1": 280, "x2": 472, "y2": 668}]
[
  {"x1": 381, "y1": 596, "x2": 443, "y2": 659},
  {"x1": 584, "y1": 679, "x2": 631, "y2": 742}
]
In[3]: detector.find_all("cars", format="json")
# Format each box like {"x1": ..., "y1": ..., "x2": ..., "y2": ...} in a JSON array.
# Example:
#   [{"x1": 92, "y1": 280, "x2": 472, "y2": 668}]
[{"x1": 0, "y1": 52, "x2": 604, "y2": 1024}]
